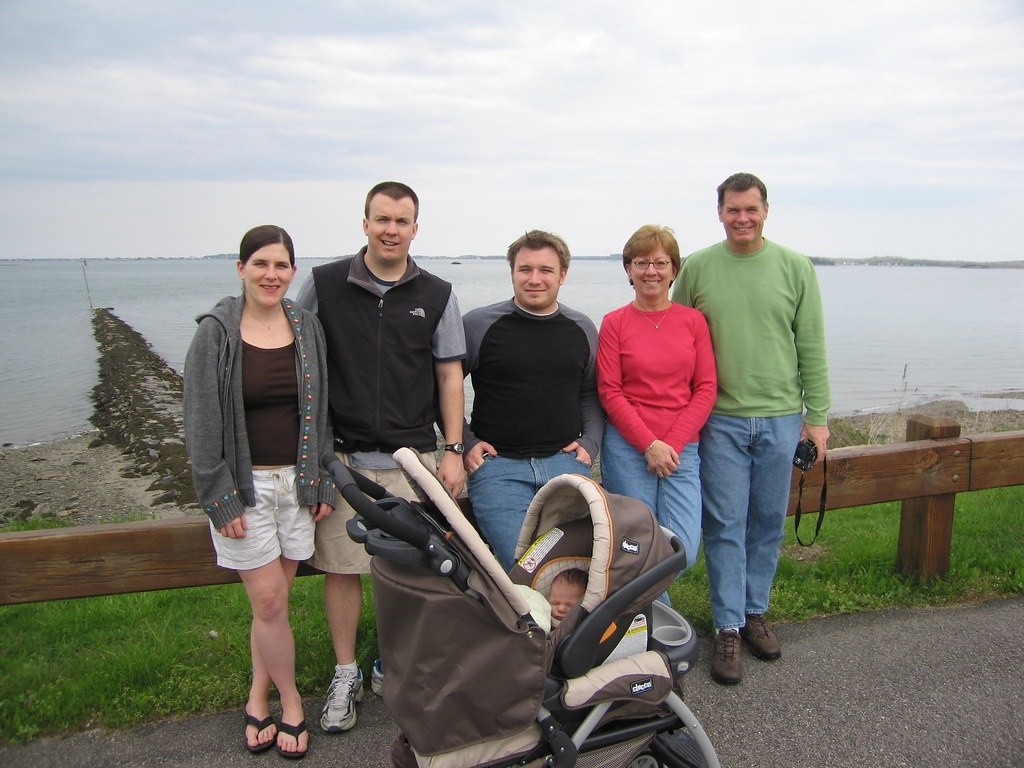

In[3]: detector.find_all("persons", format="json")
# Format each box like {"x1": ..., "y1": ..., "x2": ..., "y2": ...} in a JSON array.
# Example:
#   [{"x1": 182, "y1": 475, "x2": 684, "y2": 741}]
[
  {"x1": 183, "y1": 225, "x2": 337, "y2": 759},
  {"x1": 549, "y1": 567, "x2": 589, "y2": 621},
  {"x1": 434, "y1": 230, "x2": 607, "y2": 575},
  {"x1": 669, "y1": 174, "x2": 830, "y2": 687},
  {"x1": 294, "y1": 181, "x2": 466, "y2": 733},
  {"x1": 595, "y1": 223, "x2": 719, "y2": 608}
]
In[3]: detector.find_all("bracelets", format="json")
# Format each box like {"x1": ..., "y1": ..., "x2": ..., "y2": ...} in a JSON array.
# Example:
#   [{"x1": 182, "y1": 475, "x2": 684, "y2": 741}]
[{"x1": 648, "y1": 440, "x2": 657, "y2": 448}]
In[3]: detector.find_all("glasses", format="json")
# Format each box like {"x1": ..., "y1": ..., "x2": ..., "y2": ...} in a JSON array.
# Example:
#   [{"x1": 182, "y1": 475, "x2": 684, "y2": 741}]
[{"x1": 630, "y1": 258, "x2": 671, "y2": 270}]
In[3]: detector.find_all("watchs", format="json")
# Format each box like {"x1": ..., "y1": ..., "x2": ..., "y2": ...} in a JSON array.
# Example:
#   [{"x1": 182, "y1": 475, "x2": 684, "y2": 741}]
[{"x1": 444, "y1": 443, "x2": 466, "y2": 454}]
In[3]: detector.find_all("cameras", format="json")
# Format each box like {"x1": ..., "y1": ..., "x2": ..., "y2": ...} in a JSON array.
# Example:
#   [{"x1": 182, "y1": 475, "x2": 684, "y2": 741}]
[{"x1": 794, "y1": 438, "x2": 818, "y2": 471}]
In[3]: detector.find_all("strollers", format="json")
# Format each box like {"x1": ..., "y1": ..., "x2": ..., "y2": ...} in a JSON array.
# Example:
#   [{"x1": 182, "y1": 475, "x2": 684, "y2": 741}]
[{"x1": 319, "y1": 446, "x2": 722, "y2": 768}]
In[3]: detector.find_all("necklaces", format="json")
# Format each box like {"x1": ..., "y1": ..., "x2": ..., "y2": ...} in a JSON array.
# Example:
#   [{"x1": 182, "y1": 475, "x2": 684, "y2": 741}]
[
  {"x1": 245, "y1": 311, "x2": 273, "y2": 331},
  {"x1": 638, "y1": 305, "x2": 668, "y2": 329}
]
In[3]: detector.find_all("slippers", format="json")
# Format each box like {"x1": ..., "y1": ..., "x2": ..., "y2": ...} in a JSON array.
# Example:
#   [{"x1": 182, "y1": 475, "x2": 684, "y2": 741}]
[
  {"x1": 276, "y1": 709, "x2": 310, "y2": 759},
  {"x1": 242, "y1": 707, "x2": 279, "y2": 753}
]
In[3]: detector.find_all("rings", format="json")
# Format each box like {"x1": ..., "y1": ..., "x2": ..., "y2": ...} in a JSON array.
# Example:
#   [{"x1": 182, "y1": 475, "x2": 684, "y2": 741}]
[{"x1": 658, "y1": 471, "x2": 662, "y2": 475}]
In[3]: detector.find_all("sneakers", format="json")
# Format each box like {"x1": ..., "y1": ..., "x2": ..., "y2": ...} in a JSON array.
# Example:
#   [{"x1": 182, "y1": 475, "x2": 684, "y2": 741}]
[
  {"x1": 319, "y1": 663, "x2": 365, "y2": 733},
  {"x1": 371, "y1": 658, "x2": 384, "y2": 697},
  {"x1": 739, "y1": 613, "x2": 781, "y2": 661},
  {"x1": 710, "y1": 628, "x2": 742, "y2": 685}
]
